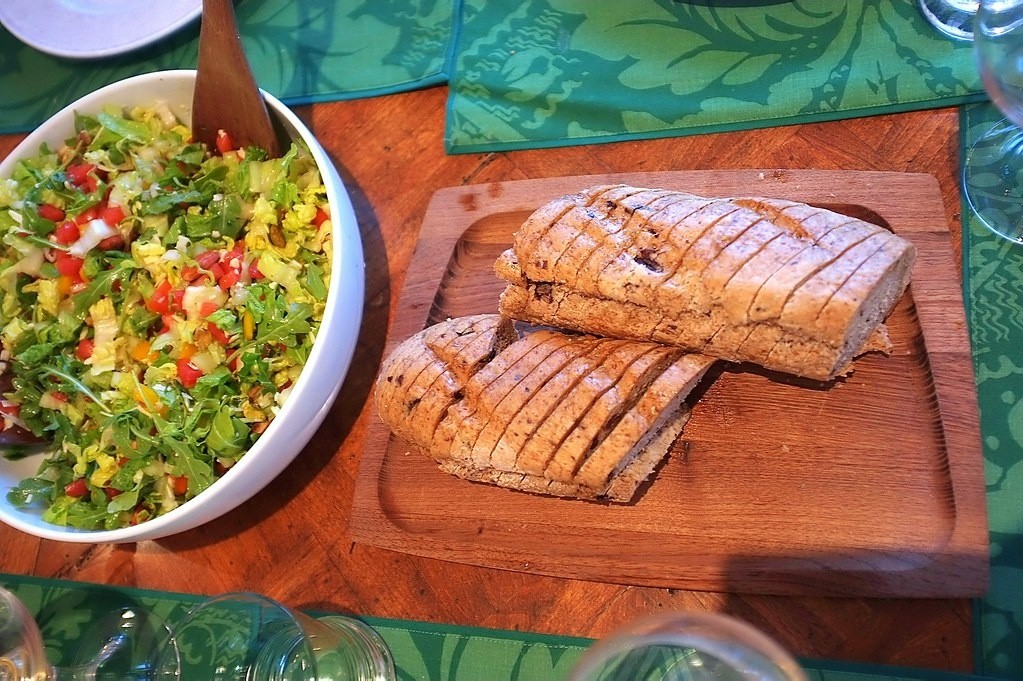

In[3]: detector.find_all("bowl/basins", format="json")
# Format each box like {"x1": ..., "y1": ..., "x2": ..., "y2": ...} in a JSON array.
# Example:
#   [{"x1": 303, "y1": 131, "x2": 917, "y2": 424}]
[{"x1": 0, "y1": 70, "x2": 365, "y2": 541}]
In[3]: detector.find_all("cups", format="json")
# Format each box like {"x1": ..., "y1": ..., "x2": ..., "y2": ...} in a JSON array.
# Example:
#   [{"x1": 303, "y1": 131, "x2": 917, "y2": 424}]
[
  {"x1": 157, "y1": 593, "x2": 395, "y2": 681},
  {"x1": 568, "y1": 607, "x2": 806, "y2": 680}
]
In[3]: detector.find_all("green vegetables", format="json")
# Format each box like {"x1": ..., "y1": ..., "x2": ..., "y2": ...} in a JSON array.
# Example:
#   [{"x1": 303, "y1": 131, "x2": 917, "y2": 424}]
[{"x1": 0, "y1": 105, "x2": 331, "y2": 531}]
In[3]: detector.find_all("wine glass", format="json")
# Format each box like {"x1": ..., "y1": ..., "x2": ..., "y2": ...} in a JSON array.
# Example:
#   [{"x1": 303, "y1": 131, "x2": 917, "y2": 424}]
[
  {"x1": 919, "y1": 0, "x2": 981, "y2": 40},
  {"x1": 0, "y1": 588, "x2": 181, "y2": 681},
  {"x1": 963, "y1": 0, "x2": 1023, "y2": 245}
]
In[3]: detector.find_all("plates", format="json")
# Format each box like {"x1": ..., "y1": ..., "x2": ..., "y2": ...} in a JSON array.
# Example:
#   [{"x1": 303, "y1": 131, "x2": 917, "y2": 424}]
[
  {"x1": 347, "y1": 170, "x2": 991, "y2": 601},
  {"x1": 0, "y1": 0, "x2": 203, "y2": 60}
]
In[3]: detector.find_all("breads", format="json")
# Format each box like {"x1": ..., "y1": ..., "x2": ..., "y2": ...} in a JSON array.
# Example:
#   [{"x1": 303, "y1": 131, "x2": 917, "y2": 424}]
[
  {"x1": 494, "y1": 180, "x2": 917, "y2": 380},
  {"x1": 372, "y1": 314, "x2": 720, "y2": 503}
]
